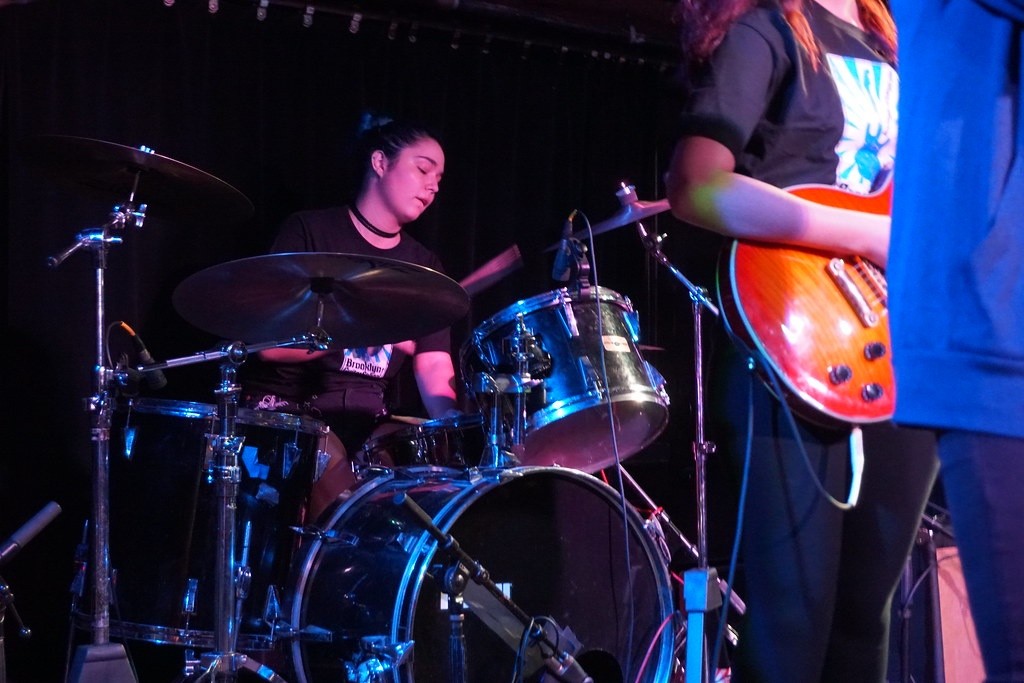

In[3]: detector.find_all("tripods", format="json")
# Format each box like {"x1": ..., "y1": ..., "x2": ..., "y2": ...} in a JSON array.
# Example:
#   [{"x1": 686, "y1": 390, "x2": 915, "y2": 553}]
[{"x1": 136, "y1": 291, "x2": 333, "y2": 683}]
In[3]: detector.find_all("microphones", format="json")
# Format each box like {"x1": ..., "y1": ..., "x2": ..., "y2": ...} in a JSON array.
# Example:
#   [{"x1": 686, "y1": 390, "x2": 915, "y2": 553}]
[
  {"x1": 120, "y1": 320, "x2": 167, "y2": 389},
  {"x1": 551, "y1": 209, "x2": 577, "y2": 281}
]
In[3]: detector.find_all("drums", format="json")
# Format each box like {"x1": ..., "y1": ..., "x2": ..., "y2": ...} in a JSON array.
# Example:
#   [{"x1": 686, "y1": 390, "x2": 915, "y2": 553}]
[
  {"x1": 457, "y1": 284, "x2": 673, "y2": 476},
  {"x1": 359, "y1": 410, "x2": 492, "y2": 475},
  {"x1": 68, "y1": 395, "x2": 331, "y2": 653},
  {"x1": 287, "y1": 462, "x2": 679, "y2": 683}
]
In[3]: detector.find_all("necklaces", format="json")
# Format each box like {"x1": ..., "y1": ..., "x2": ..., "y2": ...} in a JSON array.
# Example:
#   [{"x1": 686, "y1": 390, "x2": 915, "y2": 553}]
[{"x1": 349, "y1": 204, "x2": 402, "y2": 238}]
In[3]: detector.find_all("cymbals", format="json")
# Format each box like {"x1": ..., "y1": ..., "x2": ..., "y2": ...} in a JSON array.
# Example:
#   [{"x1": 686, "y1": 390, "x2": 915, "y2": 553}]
[
  {"x1": 18, "y1": 132, "x2": 256, "y2": 231},
  {"x1": 169, "y1": 249, "x2": 473, "y2": 352},
  {"x1": 542, "y1": 196, "x2": 670, "y2": 256}
]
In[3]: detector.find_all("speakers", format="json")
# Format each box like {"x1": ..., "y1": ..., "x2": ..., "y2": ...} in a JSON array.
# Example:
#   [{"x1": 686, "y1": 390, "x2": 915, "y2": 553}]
[{"x1": 885, "y1": 522, "x2": 988, "y2": 683}]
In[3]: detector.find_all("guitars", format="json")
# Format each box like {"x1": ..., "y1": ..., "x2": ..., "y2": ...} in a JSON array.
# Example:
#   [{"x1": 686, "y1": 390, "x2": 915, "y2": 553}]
[{"x1": 713, "y1": 168, "x2": 895, "y2": 435}]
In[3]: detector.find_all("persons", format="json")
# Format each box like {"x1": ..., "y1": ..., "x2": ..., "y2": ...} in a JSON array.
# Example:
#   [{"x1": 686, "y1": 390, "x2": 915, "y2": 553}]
[
  {"x1": 665, "y1": 0, "x2": 938, "y2": 683},
  {"x1": 886, "y1": 0, "x2": 1024, "y2": 683},
  {"x1": 256, "y1": 118, "x2": 457, "y2": 553}
]
